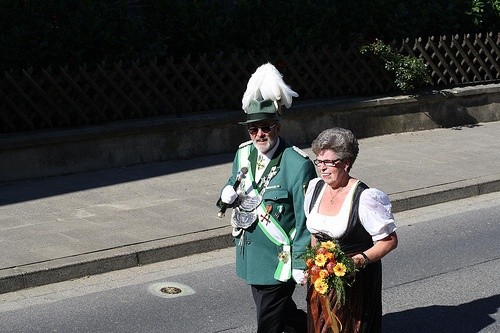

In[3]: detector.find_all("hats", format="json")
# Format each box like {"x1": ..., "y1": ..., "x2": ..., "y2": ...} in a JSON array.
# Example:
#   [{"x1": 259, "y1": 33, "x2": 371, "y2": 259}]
[{"x1": 239, "y1": 62, "x2": 299, "y2": 126}]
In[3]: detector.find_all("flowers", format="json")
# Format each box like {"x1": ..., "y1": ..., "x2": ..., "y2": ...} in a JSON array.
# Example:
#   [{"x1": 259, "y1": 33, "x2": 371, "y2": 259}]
[{"x1": 277, "y1": 240, "x2": 359, "y2": 333}]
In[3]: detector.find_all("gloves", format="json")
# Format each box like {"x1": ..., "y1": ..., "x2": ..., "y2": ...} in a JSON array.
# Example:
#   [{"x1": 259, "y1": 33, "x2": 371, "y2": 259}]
[
  {"x1": 220, "y1": 186, "x2": 238, "y2": 204},
  {"x1": 291, "y1": 269, "x2": 304, "y2": 284}
]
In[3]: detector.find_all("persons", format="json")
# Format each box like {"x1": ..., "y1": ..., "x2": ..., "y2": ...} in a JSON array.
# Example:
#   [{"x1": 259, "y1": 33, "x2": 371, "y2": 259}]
[
  {"x1": 217, "y1": 119, "x2": 318, "y2": 333},
  {"x1": 303, "y1": 127, "x2": 398, "y2": 333}
]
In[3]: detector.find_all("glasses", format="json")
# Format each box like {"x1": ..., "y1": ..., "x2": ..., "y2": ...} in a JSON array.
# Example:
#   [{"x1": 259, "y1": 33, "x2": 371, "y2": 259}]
[
  {"x1": 247, "y1": 122, "x2": 279, "y2": 134},
  {"x1": 314, "y1": 158, "x2": 342, "y2": 168}
]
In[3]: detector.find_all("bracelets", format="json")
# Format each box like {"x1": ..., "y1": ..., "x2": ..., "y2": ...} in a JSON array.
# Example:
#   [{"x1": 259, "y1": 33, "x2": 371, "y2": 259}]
[{"x1": 360, "y1": 252, "x2": 369, "y2": 263}]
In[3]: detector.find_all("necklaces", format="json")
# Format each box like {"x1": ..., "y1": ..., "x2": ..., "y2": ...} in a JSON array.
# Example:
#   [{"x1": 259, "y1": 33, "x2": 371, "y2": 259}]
[{"x1": 329, "y1": 192, "x2": 337, "y2": 204}]
